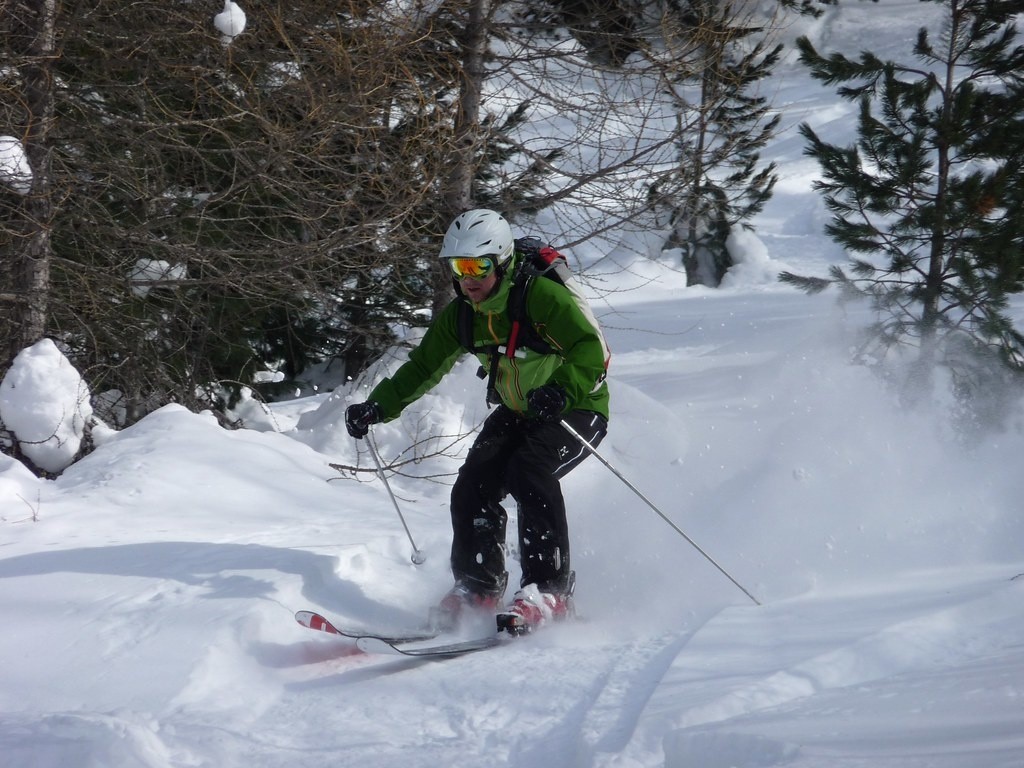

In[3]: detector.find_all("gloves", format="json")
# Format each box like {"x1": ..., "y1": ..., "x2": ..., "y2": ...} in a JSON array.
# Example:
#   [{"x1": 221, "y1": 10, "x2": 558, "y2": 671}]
[
  {"x1": 345, "y1": 401, "x2": 384, "y2": 439},
  {"x1": 527, "y1": 380, "x2": 567, "y2": 426}
]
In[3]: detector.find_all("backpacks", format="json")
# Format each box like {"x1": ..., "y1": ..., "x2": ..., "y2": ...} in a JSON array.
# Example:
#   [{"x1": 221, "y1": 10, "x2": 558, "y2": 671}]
[{"x1": 458, "y1": 234, "x2": 611, "y2": 391}]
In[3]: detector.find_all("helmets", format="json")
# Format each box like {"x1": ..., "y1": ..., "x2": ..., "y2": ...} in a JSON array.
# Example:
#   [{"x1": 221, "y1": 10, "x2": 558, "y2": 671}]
[{"x1": 439, "y1": 209, "x2": 515, "y2": 265}]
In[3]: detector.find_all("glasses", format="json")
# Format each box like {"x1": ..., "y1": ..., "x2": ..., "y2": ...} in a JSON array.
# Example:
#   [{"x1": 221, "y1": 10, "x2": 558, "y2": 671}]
[{"x1": 448, "y1": 257, "x2": 495, "y2": 281}]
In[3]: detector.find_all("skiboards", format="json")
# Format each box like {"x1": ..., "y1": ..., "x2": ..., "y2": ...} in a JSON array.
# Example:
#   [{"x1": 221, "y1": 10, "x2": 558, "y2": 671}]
[{"x1": 295, "y1": 610, "x2": 581, "y2": 661}]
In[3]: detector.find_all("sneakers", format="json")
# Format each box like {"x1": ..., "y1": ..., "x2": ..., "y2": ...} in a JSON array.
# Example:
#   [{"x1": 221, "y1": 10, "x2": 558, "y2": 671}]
[
  {"x1": 427, "y1": 571, "x2": 508, "y2": 632},
  {"x1": 496, "y1": 570, "x2": 576, "y2": 633}
]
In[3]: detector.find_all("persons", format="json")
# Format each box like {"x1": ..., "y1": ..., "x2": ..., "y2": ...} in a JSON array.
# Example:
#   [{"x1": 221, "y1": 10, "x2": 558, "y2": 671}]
[{"x1": 345, "y1": 209, "x2": 610, "y2": 633}]
